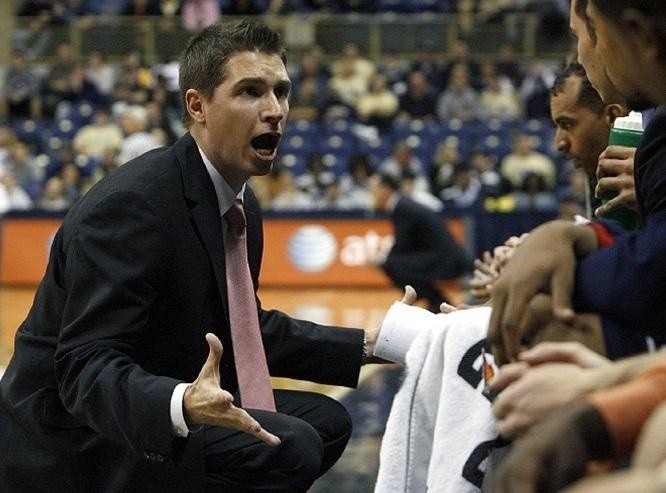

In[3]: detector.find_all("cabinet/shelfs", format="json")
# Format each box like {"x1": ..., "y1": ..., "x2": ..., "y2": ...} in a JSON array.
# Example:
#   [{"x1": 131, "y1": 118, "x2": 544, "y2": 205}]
[{"x1": 597, "y1": 109, "x2": 645, "y2": 232}]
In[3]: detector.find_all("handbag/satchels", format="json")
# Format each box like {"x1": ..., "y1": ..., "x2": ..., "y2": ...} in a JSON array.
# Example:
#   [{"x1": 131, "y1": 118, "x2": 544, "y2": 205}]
[{"x1": 360, "y1": 329, "x2": 368, "y2": 368}]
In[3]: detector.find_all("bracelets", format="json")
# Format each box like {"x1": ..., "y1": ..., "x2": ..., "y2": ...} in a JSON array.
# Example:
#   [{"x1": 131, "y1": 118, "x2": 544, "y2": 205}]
[{"x1": 360, "y1": 329, "x2": 368, "y2": 368}]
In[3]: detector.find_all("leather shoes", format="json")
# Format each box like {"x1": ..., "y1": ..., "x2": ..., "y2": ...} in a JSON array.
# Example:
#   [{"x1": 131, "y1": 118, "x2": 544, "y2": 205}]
[{"x1": 486, "y1": 401, "x2": 618, "y2": 493}]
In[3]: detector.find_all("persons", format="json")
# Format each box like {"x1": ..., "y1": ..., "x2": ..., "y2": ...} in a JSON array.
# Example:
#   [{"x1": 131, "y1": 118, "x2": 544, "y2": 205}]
[
  {"x1": 249, "y1": 3, "x2": 442, "y2": 210},
  {"x1": 0, "y1": 13, "x2": 419, "y2": 493},
  {"x1": 440, "y1": 0, "x2": 665, "y2": 492},
  {"x1": 0, "y1": 1, "x2": 224, "y2": 212}
]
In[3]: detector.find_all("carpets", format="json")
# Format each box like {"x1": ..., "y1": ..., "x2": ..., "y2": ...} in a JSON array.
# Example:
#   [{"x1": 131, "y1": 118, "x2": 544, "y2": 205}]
[{"x1": 219, "y1": 194, "x2": 279, "y2": 418}]
